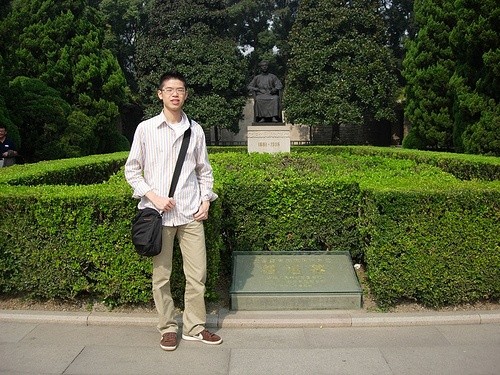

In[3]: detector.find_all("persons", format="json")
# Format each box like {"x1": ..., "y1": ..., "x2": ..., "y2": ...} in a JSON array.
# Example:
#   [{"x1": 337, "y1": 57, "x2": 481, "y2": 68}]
[
  {"x1": 0, "y1": 123, "x2": 18, "y2": 168},
  {"x1": 125, "y1": 70, "x2": 222, "y2": 351},
  {"x1": 247, "y1": 60, "x2": 283, "y2": 123}
]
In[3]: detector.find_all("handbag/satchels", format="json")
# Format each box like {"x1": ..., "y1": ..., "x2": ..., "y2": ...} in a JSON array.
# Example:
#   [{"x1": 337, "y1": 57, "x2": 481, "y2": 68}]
[{"x1": 131, "y1": 207, "x2": 162, "y2": 257}]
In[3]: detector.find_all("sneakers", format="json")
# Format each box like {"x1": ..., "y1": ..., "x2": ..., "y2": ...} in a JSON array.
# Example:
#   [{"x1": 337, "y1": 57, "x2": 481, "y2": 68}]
[
  {"x1": 159, "y1": 332, "x2": 177, "y2": 351},
  {"x1": 182, "y1": 329, "x2": 223, "y2": 345}
]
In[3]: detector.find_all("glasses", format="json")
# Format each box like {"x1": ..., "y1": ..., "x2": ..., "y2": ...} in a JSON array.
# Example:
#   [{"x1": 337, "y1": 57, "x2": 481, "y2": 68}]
[{"x1": 162, "y1": 88, "x2": 186, "y2": 94}]
